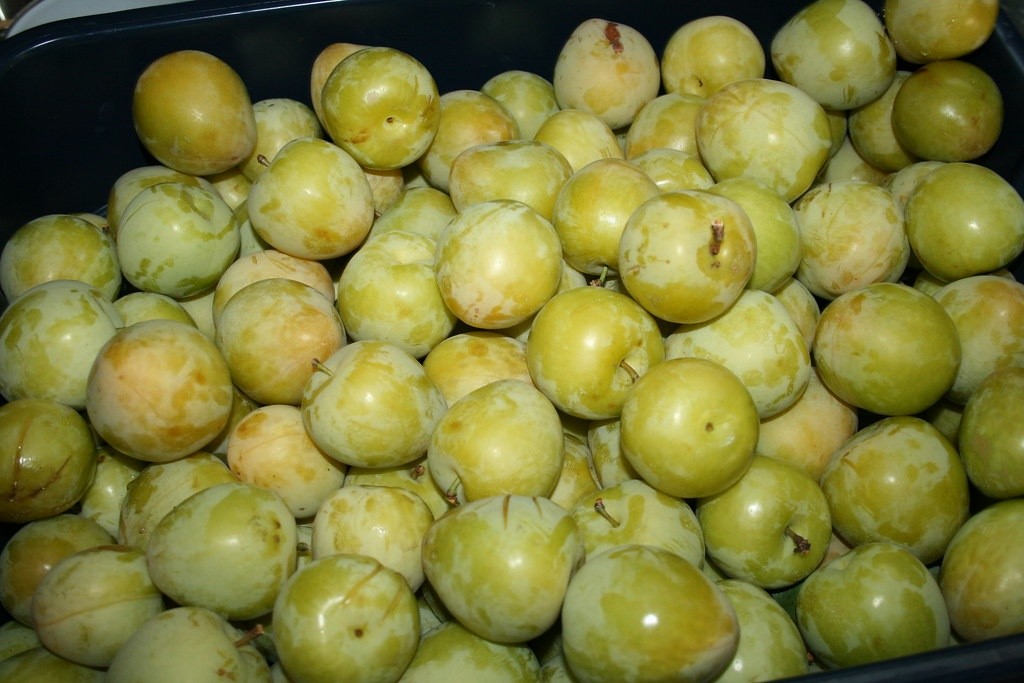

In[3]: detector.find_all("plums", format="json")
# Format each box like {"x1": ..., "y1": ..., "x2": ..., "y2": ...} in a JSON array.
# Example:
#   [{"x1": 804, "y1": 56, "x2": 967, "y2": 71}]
[{"x1": 0, "y1": 0, "x2": 1024, "y2": 683}]
